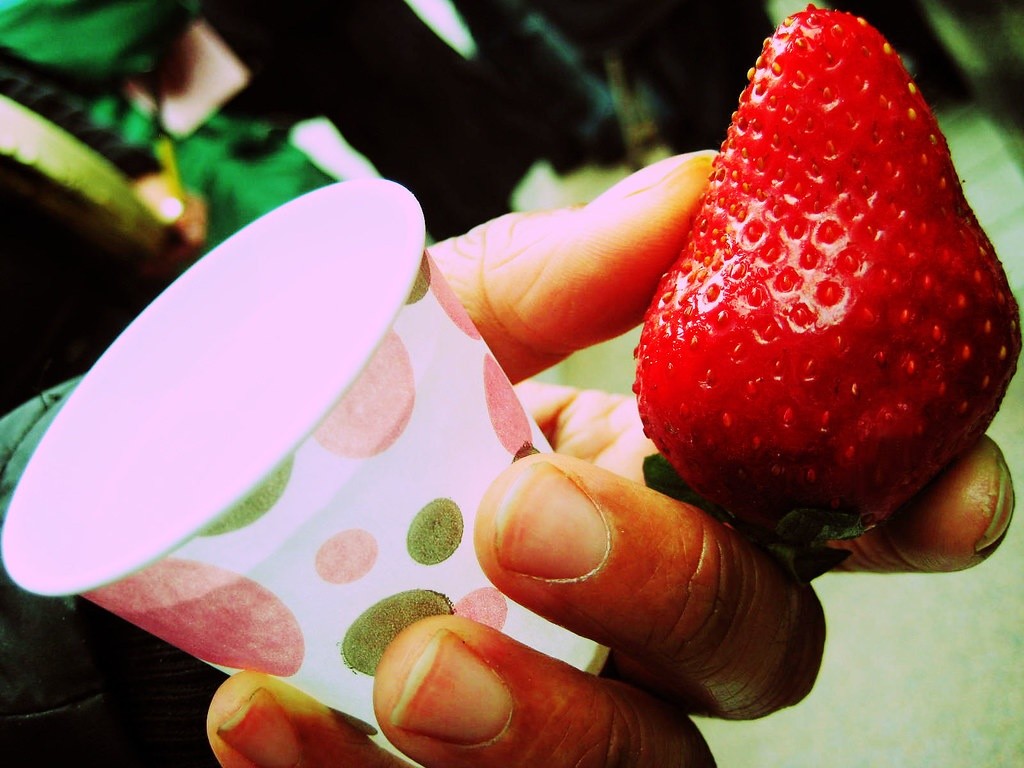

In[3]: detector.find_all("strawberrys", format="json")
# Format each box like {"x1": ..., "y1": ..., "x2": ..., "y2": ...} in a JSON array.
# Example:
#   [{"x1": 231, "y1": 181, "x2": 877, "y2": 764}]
[{"x1": 629, "y1": 3, "x2": 1024, "y2": 584}]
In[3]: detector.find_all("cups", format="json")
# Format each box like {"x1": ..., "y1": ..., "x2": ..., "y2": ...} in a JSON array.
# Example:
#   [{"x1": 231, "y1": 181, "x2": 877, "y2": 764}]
[{"x1": 0, "y1": 178, "x2": 614, "y2": 768}]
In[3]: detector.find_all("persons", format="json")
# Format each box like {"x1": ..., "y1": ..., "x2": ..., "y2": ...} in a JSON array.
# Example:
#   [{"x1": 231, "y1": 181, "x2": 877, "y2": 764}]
[{"x1": 0, "y1": 51, "x2": 1013, "y2": 768}]
